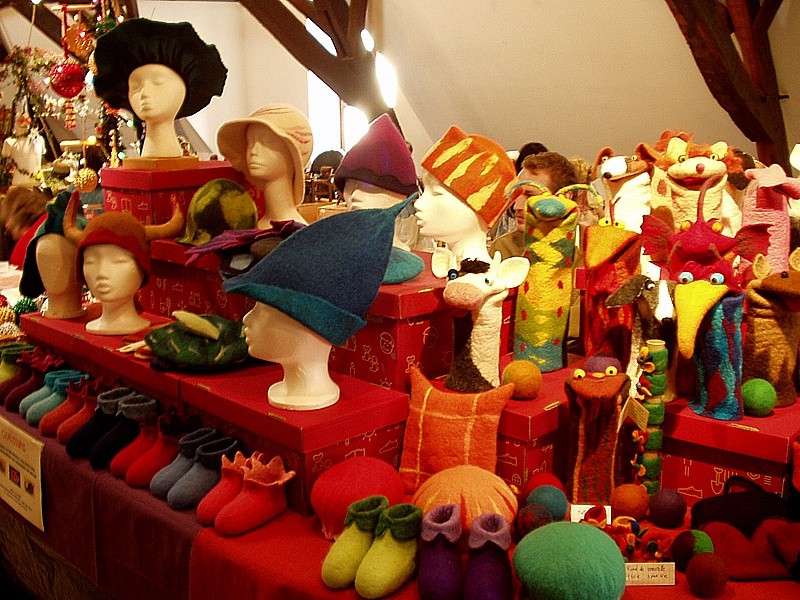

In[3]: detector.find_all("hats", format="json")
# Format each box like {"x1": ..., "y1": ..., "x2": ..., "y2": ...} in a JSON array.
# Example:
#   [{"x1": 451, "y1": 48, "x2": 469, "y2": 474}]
[
  {"x1": 216, "y1": 104, "x2": 314, "y2": 207},
  {"x1": 19, "y1": 191, "x2": 91, "y2": 299},
  {"x1": 421, "y1": 126, "x2": 525, "y2": 230},
  {"x1": 94, "y1": 20, "x2": 231, "y2": 120},
  {"x1": 63, "y1": 190, "x2": 187, "y2": 284},
  {"x1": 333, "y1": 113, "x2": 419, "y2": 197},
  {"x1": 222, "y1": 188, "x2": 421, "y2": 347}
]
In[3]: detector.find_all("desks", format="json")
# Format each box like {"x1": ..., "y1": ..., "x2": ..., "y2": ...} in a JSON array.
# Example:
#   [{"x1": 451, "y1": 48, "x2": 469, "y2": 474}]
[{"x1": 0, "y1": 404, "x2": 420, "y2": 600}]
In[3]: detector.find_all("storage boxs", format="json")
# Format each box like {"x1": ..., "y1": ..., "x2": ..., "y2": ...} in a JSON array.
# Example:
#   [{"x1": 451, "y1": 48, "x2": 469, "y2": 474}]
[{"x1": 19, "y1": 161, "x2": 800, "y2": 515}]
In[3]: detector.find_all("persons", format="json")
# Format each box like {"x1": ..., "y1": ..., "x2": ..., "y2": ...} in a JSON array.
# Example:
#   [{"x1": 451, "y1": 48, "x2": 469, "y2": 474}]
[
  {"x1": 21, "y1": 19, "x2": 520, "y2": 410},
  {"x1": 0, "y1": 186, "x2": 49, "y2": 271},
  {"x1": 489, "y1": 153, "x2": 578, "y2": 259},
  {"x1": 3, "y1": 121, "x2": 46, "y2": 188}
]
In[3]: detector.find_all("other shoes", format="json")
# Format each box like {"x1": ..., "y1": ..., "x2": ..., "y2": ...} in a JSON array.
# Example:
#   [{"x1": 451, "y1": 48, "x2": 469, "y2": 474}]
[
  {"x1": 321, "y1": 497, "x2": 389, "y2": 588},
  {"x1": 464, "y1": 513, "x2": 513, "y2": 600},
  {"x1": 355, "y1": 504, "x2": 422, "y2": 596},
  {"x1": 416, "y1": 504, "x2": 461, "y2": 600},
  {"x1": 0, "y1": 346, "x2": 296, "y2": 538}
]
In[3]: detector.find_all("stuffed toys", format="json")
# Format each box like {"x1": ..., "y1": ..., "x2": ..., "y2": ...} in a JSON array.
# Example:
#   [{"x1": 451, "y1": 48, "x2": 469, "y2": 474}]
[
  {"x1": 564, "y1": 132, "x2": 800, "y2": 526},
  {"x1": 444, "y1": 248, "x2": 530, "y2": 392},
  {"x1": 502, "y1": 182, "x2": 603, "y2": 373}
]
